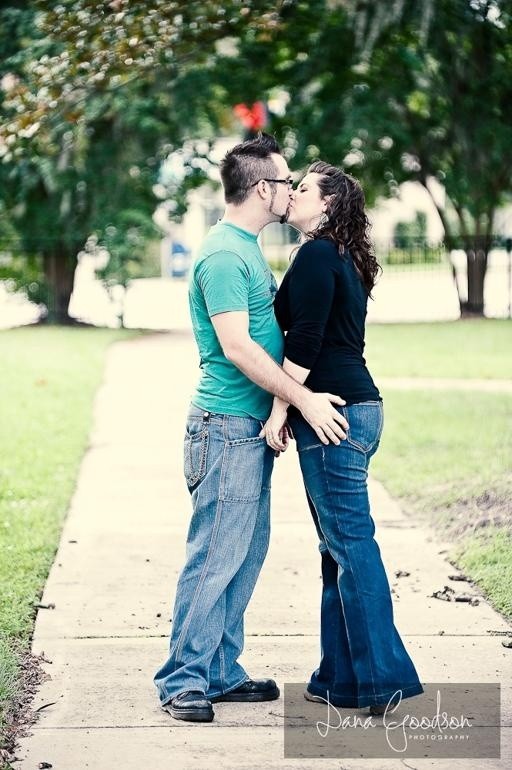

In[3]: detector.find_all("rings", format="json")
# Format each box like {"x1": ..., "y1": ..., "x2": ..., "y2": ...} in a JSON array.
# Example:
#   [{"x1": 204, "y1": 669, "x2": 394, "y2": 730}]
[{"x1": 269, "y1": 437, "x2": 273, "y2": 442}]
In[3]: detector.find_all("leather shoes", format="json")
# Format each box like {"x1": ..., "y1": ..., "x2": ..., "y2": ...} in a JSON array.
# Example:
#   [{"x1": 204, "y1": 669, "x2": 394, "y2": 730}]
[
  {"x1": 160, "y1": 691, "x2": 215, "y2": 723},
  {"x1": 211, "y1": 677, "x2": 280, "y2": 703}
]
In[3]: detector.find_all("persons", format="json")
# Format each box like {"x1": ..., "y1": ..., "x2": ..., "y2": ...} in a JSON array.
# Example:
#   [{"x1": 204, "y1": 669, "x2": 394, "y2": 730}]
[
  {"x1": 152, "y1": 138, "x2": 352, "y2": 722},
  {"x1": 260, "y1": 160, "x2": 427, "y2": 718}
]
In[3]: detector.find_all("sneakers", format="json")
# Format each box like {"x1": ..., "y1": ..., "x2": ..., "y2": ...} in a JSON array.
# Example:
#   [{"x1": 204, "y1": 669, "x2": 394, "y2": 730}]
[{"x1": 370, "y1": 699, "x2": 398, "y2": 716}]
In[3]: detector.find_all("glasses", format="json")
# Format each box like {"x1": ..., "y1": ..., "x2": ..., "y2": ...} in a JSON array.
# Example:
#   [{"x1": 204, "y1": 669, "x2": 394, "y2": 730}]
[{"x1": 251, "y1": 174, "x2": 294, "y2": 189}]
